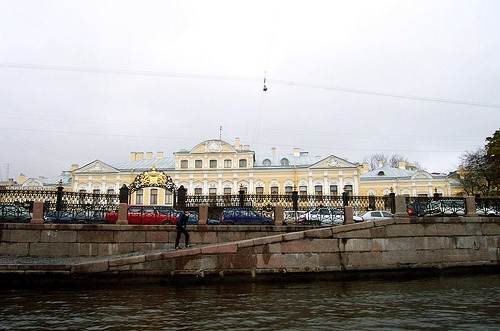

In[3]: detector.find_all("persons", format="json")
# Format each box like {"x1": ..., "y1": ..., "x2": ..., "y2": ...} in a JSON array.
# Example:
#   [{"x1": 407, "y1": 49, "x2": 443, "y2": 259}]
[{"x1": 175, "y1": 208, "x2": 193, "y2": 249}]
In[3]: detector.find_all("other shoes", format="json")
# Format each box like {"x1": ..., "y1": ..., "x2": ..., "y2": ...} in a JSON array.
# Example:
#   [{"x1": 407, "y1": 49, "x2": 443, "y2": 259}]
[
  {"x1": 186, "y1": 245, "x2": 192, "y2": 247},
  {"x1": 177, "y1": 247, "x2": 182, "y2": 249}
]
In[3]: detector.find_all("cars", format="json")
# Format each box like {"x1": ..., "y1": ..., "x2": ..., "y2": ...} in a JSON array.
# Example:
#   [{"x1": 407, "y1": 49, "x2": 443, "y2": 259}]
[
  {"x1": 423, "y1": 199, "x2": 500, "y2": 217},
  {"x1": 221, "y1": 208, "x2": 274, "y2": 224},
  {"x1": 168, "y1": 210, "x2": 220, "y2": 225},
  {"x1": 296, "y1": 207, "x2": 363, "y2": 225},
  {"x1": 359, "y1": 211, "x2": 395, "y2": 223},
  {"x1": 282, "y1": 210, "x2": 308, "y2": 221},
  {"x1": 45, "y1": 208, "x2": 107, "y2": 224},
  {"x1": 407, "y1": 201, "x2": 430, "y2": 217},
  {"x1": 106, "y1": 205, "x2": 178, "y2": 226},
  {"x1": 0, "y1": 203, "x2": 53, "y2": 222}
]
